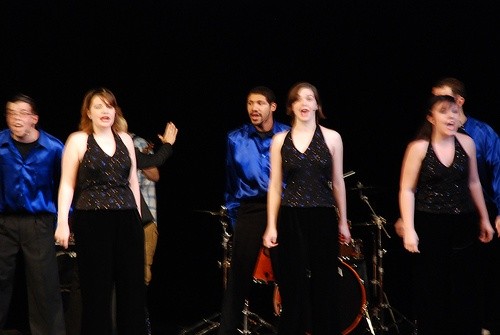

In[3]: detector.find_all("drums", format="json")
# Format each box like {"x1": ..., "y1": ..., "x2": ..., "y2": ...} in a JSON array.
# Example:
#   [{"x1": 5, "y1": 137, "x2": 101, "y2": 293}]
[
  {"x1": 273, "y1": 259, "x2": 366, "y2": 335},
  {"x1": 338, "y1": 238, "x2": 363, "y2": 260},
  {"x1": 346, "y1": 220, "x2": 352, "y2": 238}
]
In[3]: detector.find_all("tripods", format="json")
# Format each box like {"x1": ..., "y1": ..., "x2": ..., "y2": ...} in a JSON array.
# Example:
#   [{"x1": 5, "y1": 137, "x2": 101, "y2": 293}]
[{"x1": 180, "y1": 171, "x2": 419, "y2": 335}]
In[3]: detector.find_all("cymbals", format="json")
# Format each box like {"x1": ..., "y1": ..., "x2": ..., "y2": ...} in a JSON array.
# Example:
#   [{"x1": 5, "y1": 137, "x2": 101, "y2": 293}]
[{"x1": 355, "y1": 222, "x2": 377, "y2": 227}]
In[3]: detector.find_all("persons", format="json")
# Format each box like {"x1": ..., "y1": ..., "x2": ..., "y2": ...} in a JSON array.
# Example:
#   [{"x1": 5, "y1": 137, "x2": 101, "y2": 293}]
[
  {"x1": 394, "y1": 76, "x2": 500, "y2": 335},
  {"x1": 0, "y1": 94, "x2": 74, "y2": 335},
  {"x1": 118, "y1": 118, "x2": 160, "y2": 285},
  {"x1": 54, "y1": 86, "x2": 148, "y2": 335},
  {"x1": 262, "y1": 83, "x2": 351, "y2": 335},
  {"x1": 398, "y1": 95, "x2": 495, "y2": 335},
  {"x1": 115, "y1": 115, "x2": 179, "y2": 226},
  {"x1": 218, "y1": 85, "x2": 293, "y2": 335}
]
